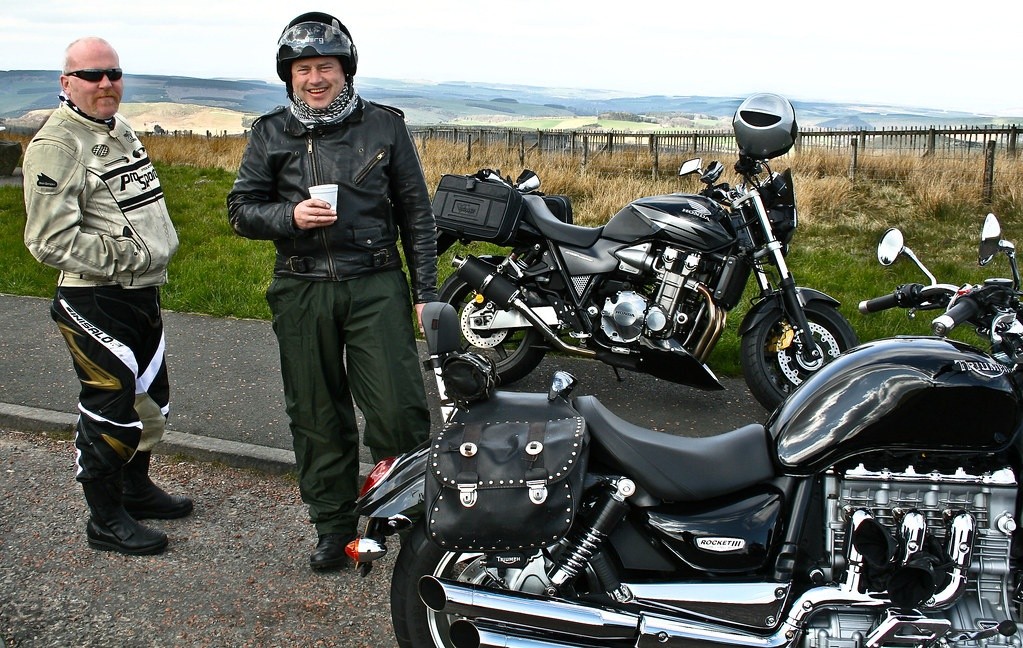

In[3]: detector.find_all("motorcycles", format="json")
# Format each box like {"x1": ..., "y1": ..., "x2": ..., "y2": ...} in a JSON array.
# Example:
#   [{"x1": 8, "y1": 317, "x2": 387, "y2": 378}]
[
  {"x1": 343, "y1": 211, "x2": 1023, "y2": 647},
  {"x1": 431, "y1": 138, "x2": 858, "y2": 422}
]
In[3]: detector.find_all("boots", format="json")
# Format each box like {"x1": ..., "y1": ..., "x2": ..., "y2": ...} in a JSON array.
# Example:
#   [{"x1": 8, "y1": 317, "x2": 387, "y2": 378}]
[
  {"x1": 82, "y1": 473, "x2": 168, "y2": 556},
  {"x1": 119, "y1": 450, "x2": 193, "y2": 519}
]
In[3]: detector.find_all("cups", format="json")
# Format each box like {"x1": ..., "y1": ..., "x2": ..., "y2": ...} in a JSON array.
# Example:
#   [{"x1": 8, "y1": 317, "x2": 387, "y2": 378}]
[{"x1": 308, "y1": 185, "x2": 339, "y2": 220}]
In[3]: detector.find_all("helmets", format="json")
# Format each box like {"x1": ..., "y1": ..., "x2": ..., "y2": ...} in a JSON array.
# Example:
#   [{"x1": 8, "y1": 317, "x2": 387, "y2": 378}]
[
  {"x1": 276, "y1": 11, "x2": 358, "y2": 82},
  {"x1": 732, "y1": 92, "x2": 797, "y2": 160}
]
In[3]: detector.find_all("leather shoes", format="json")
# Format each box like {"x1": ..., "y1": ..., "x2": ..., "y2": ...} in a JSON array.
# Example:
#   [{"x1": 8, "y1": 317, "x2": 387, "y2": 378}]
[{"x1": 310, "y1": 520, "x2": 358, "y2": 570}]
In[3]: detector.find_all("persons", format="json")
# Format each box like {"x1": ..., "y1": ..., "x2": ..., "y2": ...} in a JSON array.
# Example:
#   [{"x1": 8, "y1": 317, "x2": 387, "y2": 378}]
[
  {"x1": 227, "y1": 10, "x2": 440, "y2": 576},
  {"x1": 22, "y1": 35, "x2": 194, "y2": 557}
]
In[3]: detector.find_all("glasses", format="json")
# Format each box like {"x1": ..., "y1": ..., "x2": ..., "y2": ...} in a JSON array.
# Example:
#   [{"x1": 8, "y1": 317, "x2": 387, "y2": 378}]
[
  {"x1": 276, "y1": 22, "x2": 352, "y2": 61},
  {"x1": 64, "y1": 68, "x2": 122, "y2": 82}
]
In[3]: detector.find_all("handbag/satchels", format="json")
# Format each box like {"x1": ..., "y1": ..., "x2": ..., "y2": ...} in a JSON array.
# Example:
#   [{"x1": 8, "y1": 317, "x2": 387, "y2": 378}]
[{"x1": 425, "y1": 418, "x2": 591, "y2": 553}]
